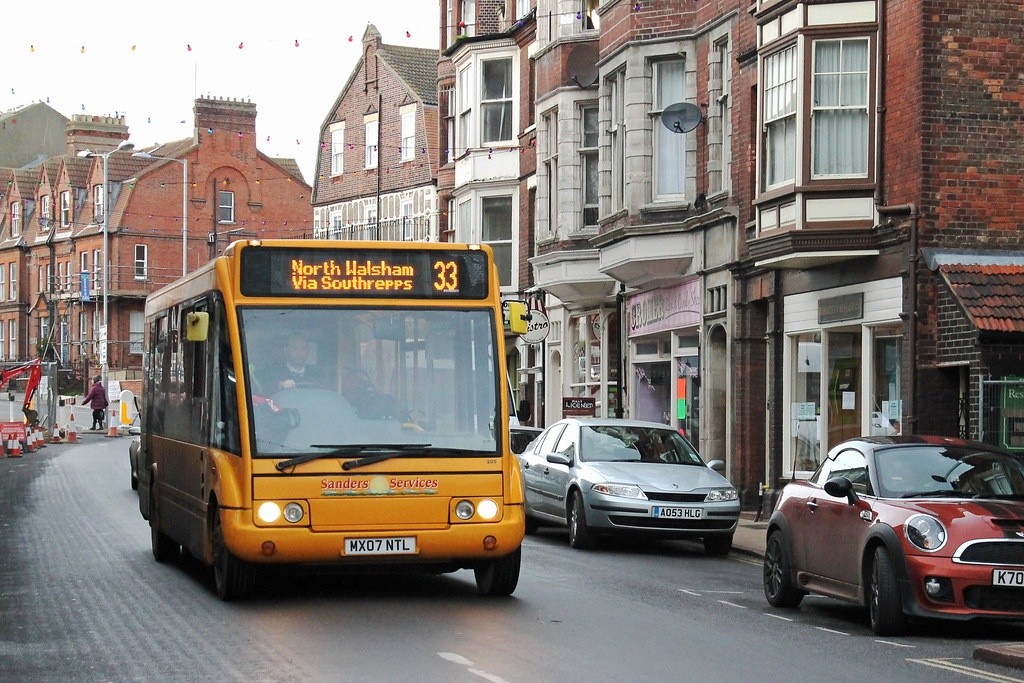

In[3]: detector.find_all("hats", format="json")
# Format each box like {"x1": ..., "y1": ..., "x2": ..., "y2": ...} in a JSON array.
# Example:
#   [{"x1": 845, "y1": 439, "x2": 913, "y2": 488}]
[{"x1": 93, "y1": 375, "x2": 101, "y2": 384}]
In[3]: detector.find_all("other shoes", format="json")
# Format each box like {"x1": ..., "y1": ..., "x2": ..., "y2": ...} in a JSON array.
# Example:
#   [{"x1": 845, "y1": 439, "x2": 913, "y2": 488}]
[
  {"x1": 90, "y1": 427, "x2": 95, "y2": 430},
  {"x1": 97, "y1": 427, "x2": 103, "y2": 430}
]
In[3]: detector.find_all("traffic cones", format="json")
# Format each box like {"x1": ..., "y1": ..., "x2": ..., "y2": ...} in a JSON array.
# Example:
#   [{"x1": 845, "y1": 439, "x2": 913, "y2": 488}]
[
  {"x1": 0, "y1": 430, "x2": 7, "y2": 459},
  {"x1": 36, "y1": 428, "x2": 47, "y2": 448},
  {"x1": 64, "y1": 413, "x2": 80, "y2": 444},
  {"x1": 104, "y1": 409, "x2": 124, "y2": 438},
  {"x1": 48, "y1": 423, "x2": 63, "y2": 444},
  {"x1": 5, "y1": 432, "x2": 23, "y2": 458},
  {"x1": 24, "y1": 431, "x2": 38, "y2": 453},
  {"x1": 30, "y1": 427, "x2": 41, "y2": 449}
]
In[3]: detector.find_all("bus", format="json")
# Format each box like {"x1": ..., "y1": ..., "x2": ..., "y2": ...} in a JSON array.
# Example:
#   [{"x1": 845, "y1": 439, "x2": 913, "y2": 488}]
[{"x1": 138, "y1": 237, "x2": 528, "y2": 605}]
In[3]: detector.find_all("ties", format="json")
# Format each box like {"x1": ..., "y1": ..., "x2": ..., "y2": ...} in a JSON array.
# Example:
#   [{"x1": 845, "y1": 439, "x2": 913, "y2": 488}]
[{"x1": 293, "y1": 371, "x2": 303, "y2": 384}]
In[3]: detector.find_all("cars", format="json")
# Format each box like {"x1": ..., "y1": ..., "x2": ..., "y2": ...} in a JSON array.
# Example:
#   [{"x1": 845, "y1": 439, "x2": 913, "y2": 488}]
[
  {"x1": 129, "y1": 425, "x2": 141, "y2": 489},
  {"x1": 514, "y1": 418, "x2": 741, "y2": 558},
  {"x1": 764, "y1": 434, "x2": 1024, "y2": 636}
]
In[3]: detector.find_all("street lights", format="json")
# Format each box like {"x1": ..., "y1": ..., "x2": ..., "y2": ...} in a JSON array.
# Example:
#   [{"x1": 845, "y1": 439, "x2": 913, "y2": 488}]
[
  {"x1": 130, "y1": 151, "x2": 188, "y2": 280},
  {"x1": 76, "y1": 140, "x2": 135, "y2": 431}
]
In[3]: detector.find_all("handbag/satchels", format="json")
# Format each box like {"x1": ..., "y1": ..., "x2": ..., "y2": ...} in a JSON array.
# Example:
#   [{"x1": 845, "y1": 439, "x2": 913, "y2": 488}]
[{"x1": 99, "y1": 409, "x2": 104, "y2": 419}]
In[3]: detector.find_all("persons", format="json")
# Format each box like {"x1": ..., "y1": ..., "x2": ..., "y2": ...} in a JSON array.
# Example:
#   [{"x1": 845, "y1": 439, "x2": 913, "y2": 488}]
[
  {"x1": 81, "y1": 377, "x2": 108, "y2": 431},
  {"x1": 269, "y1": 328, "x2": 332, "y2": 391}
]
